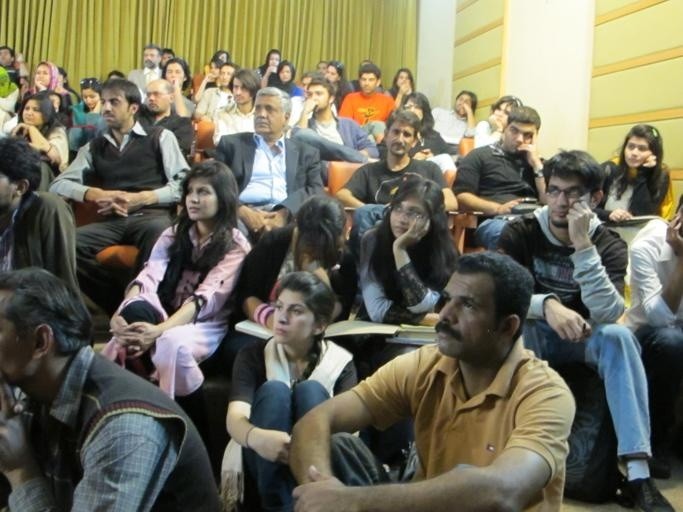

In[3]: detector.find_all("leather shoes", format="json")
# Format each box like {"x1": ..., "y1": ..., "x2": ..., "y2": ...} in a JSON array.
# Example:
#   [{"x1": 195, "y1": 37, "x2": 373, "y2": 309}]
[
  {"x1": 647, "y1": 454, "x2": 671, "y2": 479},
  {"x1": 614, "y1": 477, "x2": 675, "y2": 512}
]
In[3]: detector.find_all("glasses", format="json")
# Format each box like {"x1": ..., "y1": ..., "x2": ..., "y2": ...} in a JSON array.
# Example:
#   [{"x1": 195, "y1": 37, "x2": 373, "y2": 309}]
[
  {"x1": 545, "y1": 185, "x2": 582, "y2": 198},
  {"x1": 80, "y1": 78, "x2": 97, "y2": 85},
  {"x1": 388, "y1": 206, "x2": 423, "y2": 223}
]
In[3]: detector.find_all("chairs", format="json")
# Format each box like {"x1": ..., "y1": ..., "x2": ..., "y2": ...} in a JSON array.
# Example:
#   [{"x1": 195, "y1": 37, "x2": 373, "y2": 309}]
[
  {"x1": 457, "y1": 138, "x2": 478, "y2": 157},
  {"x1": 445, "y1": 170, "x2": 492, "y2": 254},
  {"x1": 71, "y1": 181, "x2": 143, "y2": 267},
  {"x1": 328, "y1": 162, "x2": 368, "y2": 240},
  {"x1": 190, "y1": 117, "x2": 217, "y2": 167}
]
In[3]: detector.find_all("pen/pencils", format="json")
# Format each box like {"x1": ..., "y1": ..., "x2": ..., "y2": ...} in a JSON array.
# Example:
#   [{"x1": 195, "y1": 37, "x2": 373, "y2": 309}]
[
  {"x1": 325, "y1": 264, "x2": 341, "y2": 273},
  {"x1": 583, "y1": 323, "x2": 586, "y2": 333}
]
